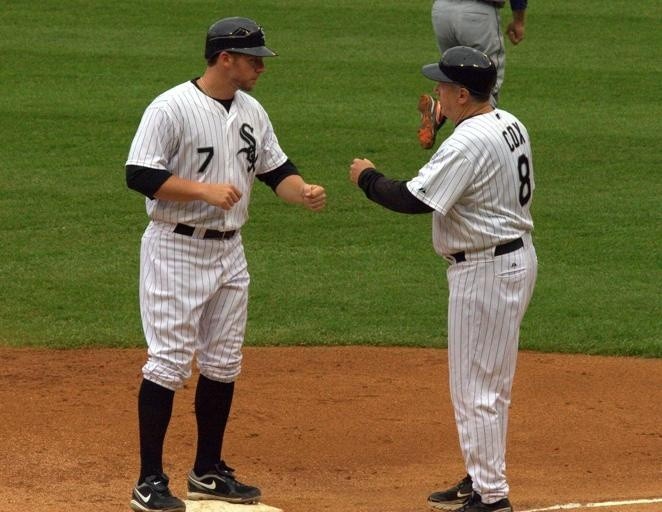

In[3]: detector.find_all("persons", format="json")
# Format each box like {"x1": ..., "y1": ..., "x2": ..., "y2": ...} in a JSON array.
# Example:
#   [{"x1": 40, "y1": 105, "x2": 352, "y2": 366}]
[
  {"x1": 125, "y1": 17, "x2": 326, "y2": 512},
  {"x1": 350, "y1": 46, "x2": 537, "y2": 512},
  {"x1": 417, "y1": 0, "x2": 527, "y2": 150}
]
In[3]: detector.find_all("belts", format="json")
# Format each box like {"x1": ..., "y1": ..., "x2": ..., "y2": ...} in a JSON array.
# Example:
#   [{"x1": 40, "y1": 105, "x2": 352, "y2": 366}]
[
  {"x1": 153, "y1": 219, "x2": 236, "y2": 239},
  {"x1": 447, "y1": 237, "x2": 524, "y2": 265}
]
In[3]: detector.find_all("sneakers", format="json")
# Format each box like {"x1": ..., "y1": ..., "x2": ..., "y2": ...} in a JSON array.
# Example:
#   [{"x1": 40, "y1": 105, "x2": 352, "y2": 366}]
[
  {"x1": 416, "y1": 94, "x2": 448, "y2": 150},
  {"x1": 427, "y1": 473, "x2": 512, "y2": 512},
  {"x1": 130, "y1": 461, "x2": 262, "y2": 512}
]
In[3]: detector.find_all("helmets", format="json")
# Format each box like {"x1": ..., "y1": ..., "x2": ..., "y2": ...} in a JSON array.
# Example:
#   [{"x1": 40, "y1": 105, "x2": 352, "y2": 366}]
[
  {"x1": 205, "y1": 17, "x2": 279, "y2": 59},
  {"x1": 422, "y1": 45, "x2": 497, "y2": 89}
]
